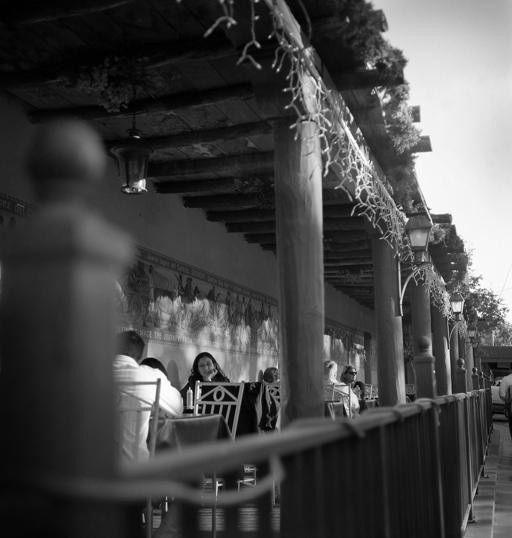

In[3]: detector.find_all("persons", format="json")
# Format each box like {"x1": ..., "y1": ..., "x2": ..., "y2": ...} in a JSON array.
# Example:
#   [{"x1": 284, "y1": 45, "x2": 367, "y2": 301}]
[
  {"x1": 113, "y1": 330, "x2": 365, "y2": 473},
  {"x1": 500, "y1": 362, "x2": 512, "y2": 436}
]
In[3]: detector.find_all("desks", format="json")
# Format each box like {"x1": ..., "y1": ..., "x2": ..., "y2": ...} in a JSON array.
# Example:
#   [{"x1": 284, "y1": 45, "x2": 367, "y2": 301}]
[{"x1": 155, "y1": 413, "x2": 220, "y2": 512}]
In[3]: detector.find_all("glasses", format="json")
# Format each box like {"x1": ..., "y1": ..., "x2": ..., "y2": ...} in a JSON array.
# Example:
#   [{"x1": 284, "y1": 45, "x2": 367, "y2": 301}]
[{"x1": 349, "y1": 371, "x2": 357, "y2": 375}]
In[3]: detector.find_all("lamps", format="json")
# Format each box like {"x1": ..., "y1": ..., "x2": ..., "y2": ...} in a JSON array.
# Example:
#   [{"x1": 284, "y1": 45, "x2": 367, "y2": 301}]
[
  {"x1": 401, "y1": 202, "x2": 430, "y2": 268},
  {"x1": 109, "y1": 81, "x2": 151, "y2": 195},
  {"x1": 448, "y1": 288, "x2": 478, "y2": 346}
]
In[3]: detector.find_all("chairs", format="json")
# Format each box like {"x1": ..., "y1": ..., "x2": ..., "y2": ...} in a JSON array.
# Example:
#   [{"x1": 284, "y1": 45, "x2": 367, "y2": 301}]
[
  {"x1": 264, "y1": 382, "x2": 417, "y2": 431},
  {"x1": 112, "y1": 381, "x2": 162, "y2": 536},
  {"x1": 186, "y1": 380, "x2": 244, "y2": 497}
]
